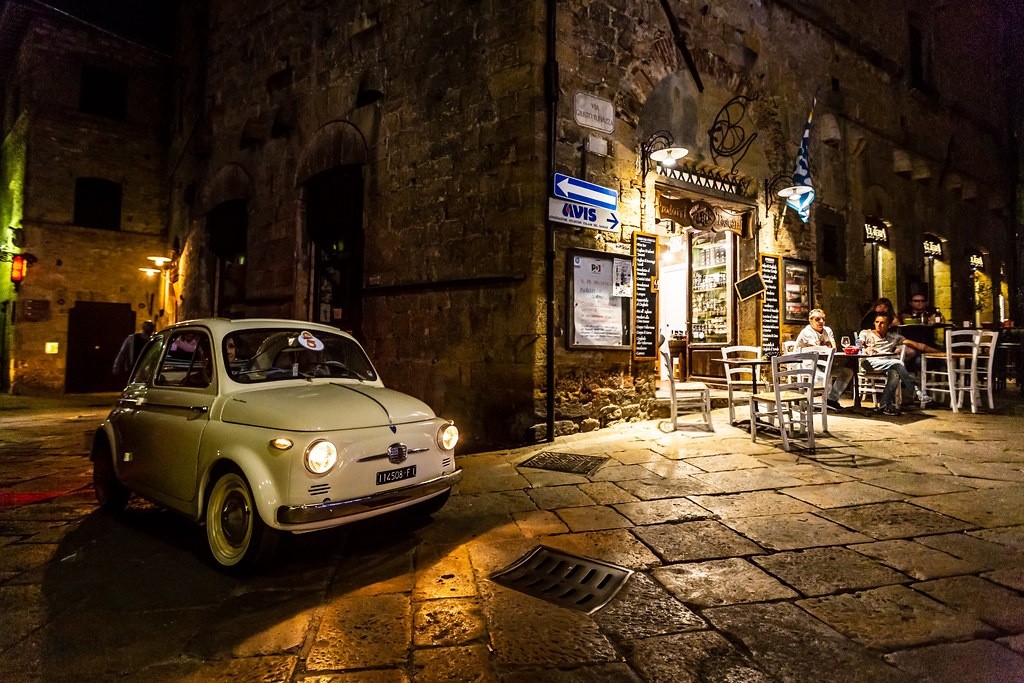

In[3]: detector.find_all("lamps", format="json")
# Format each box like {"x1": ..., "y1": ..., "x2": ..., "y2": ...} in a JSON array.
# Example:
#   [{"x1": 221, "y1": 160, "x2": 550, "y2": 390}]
[
  {"x1": 138, "y1": 244, "x2": 178, "y2": 277},
  {"x1": 766, "y1": 171, "x2": 813, "y2": 218},
  {"x1": 640, "y1": 130, "x2": 690, "y2": 187}
]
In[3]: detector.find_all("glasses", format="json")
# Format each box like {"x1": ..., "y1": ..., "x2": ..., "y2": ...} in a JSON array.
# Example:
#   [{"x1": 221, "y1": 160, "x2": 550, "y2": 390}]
[
  {"x1": 815, "y1": 317, "x2": 827, "y2": 322},
  {"x1": 912, "y1": 299, "x2": 927, "y2": 303}
]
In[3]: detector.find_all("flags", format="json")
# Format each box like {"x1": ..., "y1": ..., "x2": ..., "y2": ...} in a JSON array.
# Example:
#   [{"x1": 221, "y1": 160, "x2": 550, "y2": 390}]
[{"x1": 787, "y1": 123, "x2": 815, "y2": 222}]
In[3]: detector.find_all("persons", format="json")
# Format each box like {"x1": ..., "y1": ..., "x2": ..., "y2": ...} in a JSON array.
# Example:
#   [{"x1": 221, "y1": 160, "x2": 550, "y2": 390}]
[
  {"x1": 860, "y1": 298, "x2": 900, "y2": 330},
  {"x1": 793, "y1": 309, "x2": 853, "y2": 411},
  {"x1": 900, "y1": 293, "x2": 945, "y2": 370},
  {"x1": 181, "y1": 337, "x2": 250, "y2": 386},
  {"x1": 171, "y1": 333, "x2": 196, "y2": 360},
  {"x1": 112, "y1": 320, "x2": 155, "y2": 373},
  {"x1": 858, "y1": 313, "x2": 941, "y2": 415}
]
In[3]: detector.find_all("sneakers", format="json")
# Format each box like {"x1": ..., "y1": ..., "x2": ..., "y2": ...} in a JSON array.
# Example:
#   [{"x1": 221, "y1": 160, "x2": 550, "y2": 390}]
[
  {"x1": 827, "y1": 398, "x2": 844, "y2": 411},
  {"x1": 912, "y1": 386, "x2": 933, "y2": 403},
  {"x1": 879, "y1": 403, "x2": 901, "y2": 415}
]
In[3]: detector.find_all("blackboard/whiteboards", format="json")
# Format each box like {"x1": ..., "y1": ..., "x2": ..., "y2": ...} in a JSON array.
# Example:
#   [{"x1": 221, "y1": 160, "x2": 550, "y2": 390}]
[
  {"x1": 759, "y1": 252, "x2": 783, "y2": 356},
  {"x1": 735, "y1": 271, "x2": 768, "y2": 302},
  {"x1": 632, "y1": 231, "x2": 658, "y2": 361}
]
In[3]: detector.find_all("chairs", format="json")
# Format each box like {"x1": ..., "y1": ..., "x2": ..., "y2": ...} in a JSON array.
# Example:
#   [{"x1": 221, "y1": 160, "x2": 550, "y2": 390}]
[
  {"x1": 661, "y1": 349, "x2": 712, "y2": 432},
  {"x1": 720, "y1": 340, "x2": 836, "y2": 451},
  {"x1": 854, "y1": 328, "x2": 1024, "y2": 414}
]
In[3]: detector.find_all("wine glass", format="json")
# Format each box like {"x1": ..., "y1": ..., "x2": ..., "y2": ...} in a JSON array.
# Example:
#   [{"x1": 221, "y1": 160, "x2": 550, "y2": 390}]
[
  {"x1": 841, "y1": 336, "x2": 850, "y2": 354},
  {"x1": 867, "y1": 336, "x2": 875, "y2": 355}
]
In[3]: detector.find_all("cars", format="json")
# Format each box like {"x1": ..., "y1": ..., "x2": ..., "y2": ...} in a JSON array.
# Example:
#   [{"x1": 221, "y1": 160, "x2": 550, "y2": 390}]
[{"x1": 89, "y1": 318, "x2": 463, "y2": 574}]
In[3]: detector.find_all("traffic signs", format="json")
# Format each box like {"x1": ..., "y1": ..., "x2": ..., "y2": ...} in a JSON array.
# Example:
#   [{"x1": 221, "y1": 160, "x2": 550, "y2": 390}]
[{"x1": 549, "y1": 197, "x2": 622, "y2": 233}]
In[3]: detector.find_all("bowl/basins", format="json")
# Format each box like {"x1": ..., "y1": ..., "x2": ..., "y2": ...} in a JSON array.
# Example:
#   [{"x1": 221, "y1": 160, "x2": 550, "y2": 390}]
[{"x1": 845, "y1": 348, "x2": 860, "y2": 354}]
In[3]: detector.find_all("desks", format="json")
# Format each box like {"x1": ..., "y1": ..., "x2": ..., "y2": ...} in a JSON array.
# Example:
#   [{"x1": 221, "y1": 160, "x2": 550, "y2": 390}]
[
  {"x1": 711, "y1": 357, "x2": 803, "y2": 433},
  {"x1": 668, "y1": 339, "x2": 687, "y2": 384},
  {"x1": 892, "y1": 322, "x2": 956, "y2": 380},
  {"x1": 819, "y1": 351, "x2": 897, "y2": 417}
]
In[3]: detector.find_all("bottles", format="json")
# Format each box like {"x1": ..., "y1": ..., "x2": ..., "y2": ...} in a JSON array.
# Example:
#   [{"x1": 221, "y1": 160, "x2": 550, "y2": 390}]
[
  {"x1": 935, "y1": 309, "x2": 941, "y2": 324},
  {"x1": 855, "y1": 337, "x2": 862, "y2": 354},
  {"x1": 691, "y1": 246, "x2": 727, "y2": 343}
]
